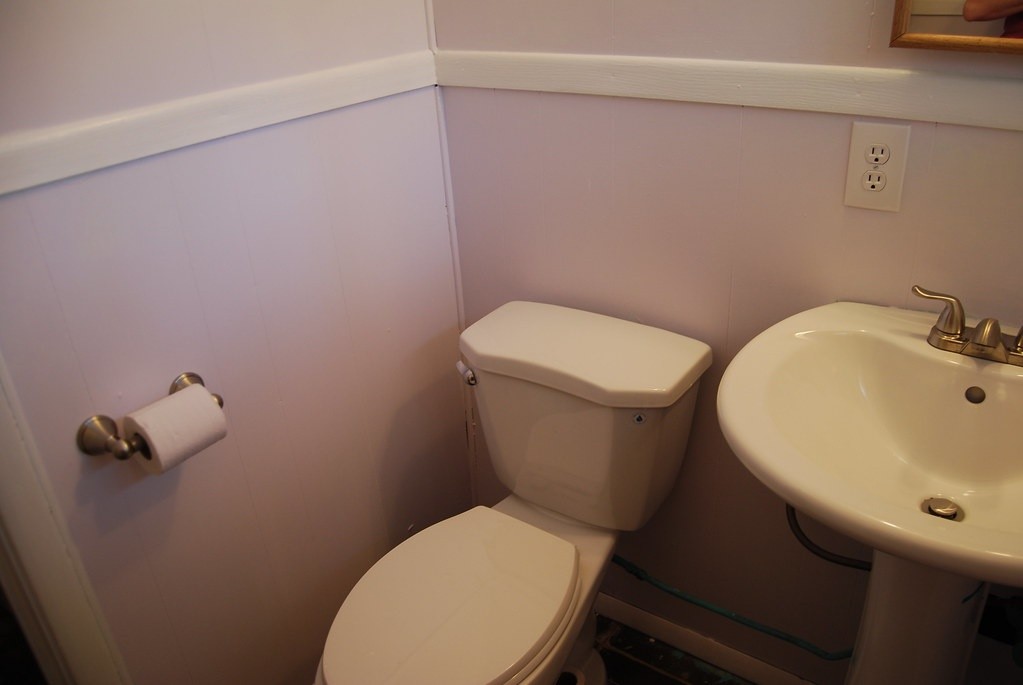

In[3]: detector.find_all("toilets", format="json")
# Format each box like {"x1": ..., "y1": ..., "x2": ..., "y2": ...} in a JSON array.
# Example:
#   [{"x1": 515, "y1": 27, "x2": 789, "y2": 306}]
[{"x1": 310, "y1": 296, "x2": 712, "y2": 685}]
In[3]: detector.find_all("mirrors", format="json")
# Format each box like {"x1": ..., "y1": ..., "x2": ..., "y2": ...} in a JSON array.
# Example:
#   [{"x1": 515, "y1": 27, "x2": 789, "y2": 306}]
[{"x1": 886, "y1": 0, "x2": 1023, "y2": 55}]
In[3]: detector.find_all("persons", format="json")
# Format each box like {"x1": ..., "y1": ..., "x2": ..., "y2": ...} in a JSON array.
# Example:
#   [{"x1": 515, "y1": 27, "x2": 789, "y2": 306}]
[{"x1": 963, "y1": 0, "x2": 1023, "y2": 39}]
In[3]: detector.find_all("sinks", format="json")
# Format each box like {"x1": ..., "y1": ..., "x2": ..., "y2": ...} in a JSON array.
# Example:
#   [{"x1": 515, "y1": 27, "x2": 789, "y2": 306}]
[{"x1": 712, "y1": 299, "x2": 1023, "y2": 591}]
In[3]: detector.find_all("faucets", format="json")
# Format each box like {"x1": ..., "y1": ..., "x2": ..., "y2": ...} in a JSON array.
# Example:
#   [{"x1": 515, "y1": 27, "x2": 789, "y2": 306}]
[{"x1": 967, "y1": 315, "x2": 1011, "y2": 366}]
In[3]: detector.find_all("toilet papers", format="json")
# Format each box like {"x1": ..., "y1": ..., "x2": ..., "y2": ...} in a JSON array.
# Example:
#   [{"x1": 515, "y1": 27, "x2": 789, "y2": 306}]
[{"x1": 122, "y1": 378, "x2": 233, "y2": 477}]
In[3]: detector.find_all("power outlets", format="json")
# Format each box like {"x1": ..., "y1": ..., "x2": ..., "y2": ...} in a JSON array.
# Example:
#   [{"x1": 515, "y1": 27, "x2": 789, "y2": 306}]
[{"x1": 843, "y1": 121, "x2": 911, "y2": 212}]
